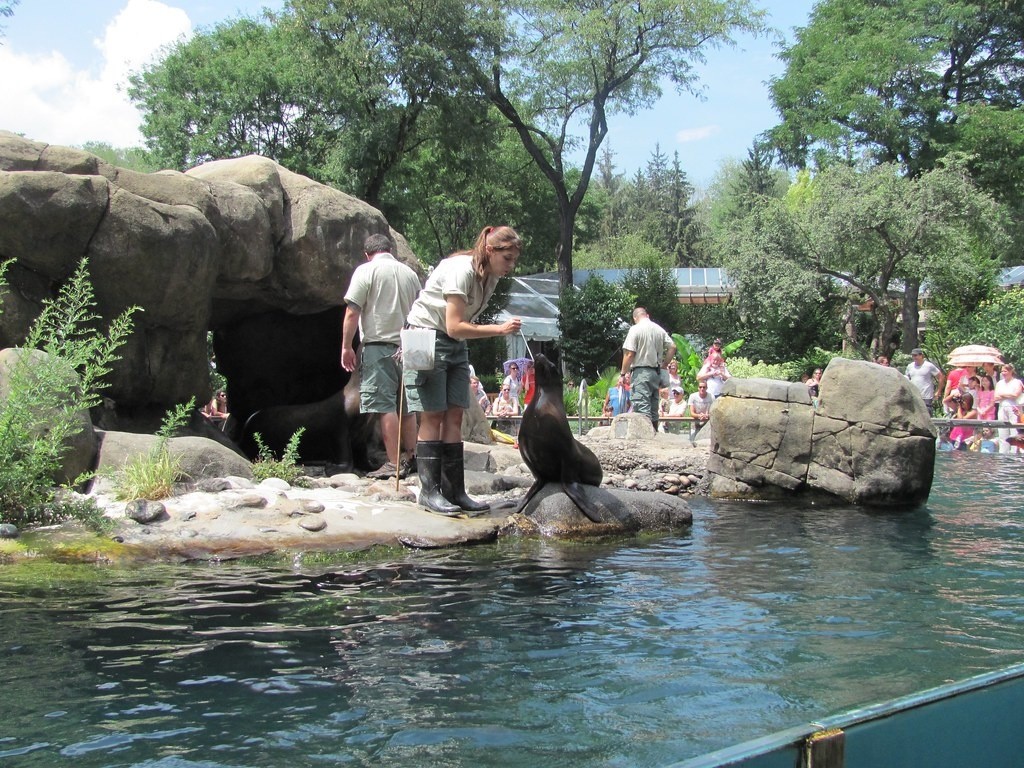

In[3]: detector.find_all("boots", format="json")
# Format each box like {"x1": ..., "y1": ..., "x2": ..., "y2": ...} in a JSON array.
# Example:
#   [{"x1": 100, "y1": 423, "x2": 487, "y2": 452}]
[
  {"x1": 440, "y1": 442, "x2": 491, "y2": 513},
  {"x1": 416, "y1": 440, "x2": 462, "y2": 517}
]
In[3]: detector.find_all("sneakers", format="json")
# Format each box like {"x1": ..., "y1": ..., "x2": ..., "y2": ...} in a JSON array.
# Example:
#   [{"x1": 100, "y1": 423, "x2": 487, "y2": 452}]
[
  {"x1": 366, "y1": 462, "x2": 405, "y2": 479},
  {"x1": 401, "y1": 458, "x2": 417, "y2": 474}
]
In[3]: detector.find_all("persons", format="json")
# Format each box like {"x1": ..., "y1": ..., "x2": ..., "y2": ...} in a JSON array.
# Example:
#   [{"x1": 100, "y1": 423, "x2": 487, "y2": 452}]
[
  {"x1": 801, "y1": 368, "x2": 823, "y2": 407},
  {"x1": 400, "y1": 227, "x2": 535, "y2": 515},
  {"x1": 601, "y1": 340, "x2": 732, "y2": 440},
  {"x1": 877, "y1": 349, "x2": 1024, "y2": 454},
  {"x1": 201, "y1": 391, "x2": 228, "y2": 419},
  {"x1": 341, "y1": 234, "x2": 423, "y2": 480},
  {"x1": 618, "y1": 308, "x2": 676, "y2": 433}
]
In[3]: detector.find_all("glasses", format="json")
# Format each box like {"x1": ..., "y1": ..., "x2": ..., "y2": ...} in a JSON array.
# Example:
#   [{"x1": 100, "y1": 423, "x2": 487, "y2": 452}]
[
  {"x1": 220, "y1": 395, "x2": 224, "y2": 397},
  {"x1": 699, "y1": 386, "x2": 706, "y2": 388},
  {"x1": 503, "y1": 389, "x2": 510, "y2": 391},
  {"x1": 673, "y1": 393, "x2": 680, "y2": 395},
  {"x1": 885, "y1": 361, "x2": 889, "y2": 365},
  {"x1": 511, "y1": 368, "x2": 518, "y2": 370}
]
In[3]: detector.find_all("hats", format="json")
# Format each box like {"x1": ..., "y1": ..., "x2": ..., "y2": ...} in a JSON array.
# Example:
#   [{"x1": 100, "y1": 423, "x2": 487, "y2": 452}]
[
  {"x1": 714, "y1": 339, "x2": 721, "y2": 345},
  {"x1": 672, "y1": 387, "x2": 684, "y2": 395},
  {"x1": 910, "y1": 349, "x2": 922, "y2": 355}
]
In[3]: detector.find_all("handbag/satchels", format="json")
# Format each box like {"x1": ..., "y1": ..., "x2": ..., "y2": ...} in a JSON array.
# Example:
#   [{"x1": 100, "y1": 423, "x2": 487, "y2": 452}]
[
  {"x1": 491, "y1": 420, "x2": 497, "y2": 429},
  {"x1": 399, "y1": 322, "x2": 436, "y2": 370},
  {"x1": 810, "y1": 380, "x2": 818, "y2": 397}
]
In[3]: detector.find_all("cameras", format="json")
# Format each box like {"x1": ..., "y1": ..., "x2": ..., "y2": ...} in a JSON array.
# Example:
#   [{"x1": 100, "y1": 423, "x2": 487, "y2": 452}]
[{"x1": 953, "y1": 397, "x2": 963, "y2": 403}]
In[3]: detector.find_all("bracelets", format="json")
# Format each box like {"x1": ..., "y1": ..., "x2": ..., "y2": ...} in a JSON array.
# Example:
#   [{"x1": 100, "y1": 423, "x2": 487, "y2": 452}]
[{"x1": 620, "y1": 372, "x2": 625, "y2": 376}]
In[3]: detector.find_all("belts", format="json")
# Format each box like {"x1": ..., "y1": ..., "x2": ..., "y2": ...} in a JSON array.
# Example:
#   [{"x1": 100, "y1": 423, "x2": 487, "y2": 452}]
[
  {"x1": 630, "y1": 366, "x2": 658, "y2": 372},
  {"x1": 363, "y1": 341, "x2": 400, "y2": 349}
]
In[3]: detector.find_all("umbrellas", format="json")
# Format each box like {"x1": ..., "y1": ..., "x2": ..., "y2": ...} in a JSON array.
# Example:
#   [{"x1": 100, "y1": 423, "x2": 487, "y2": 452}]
[
  {"x1": 946, "y1": 345, "x2": 1004, "y2": 377},
  {"x1": 503, "y1": 357, "x2": 534, "y2": 382}
]
